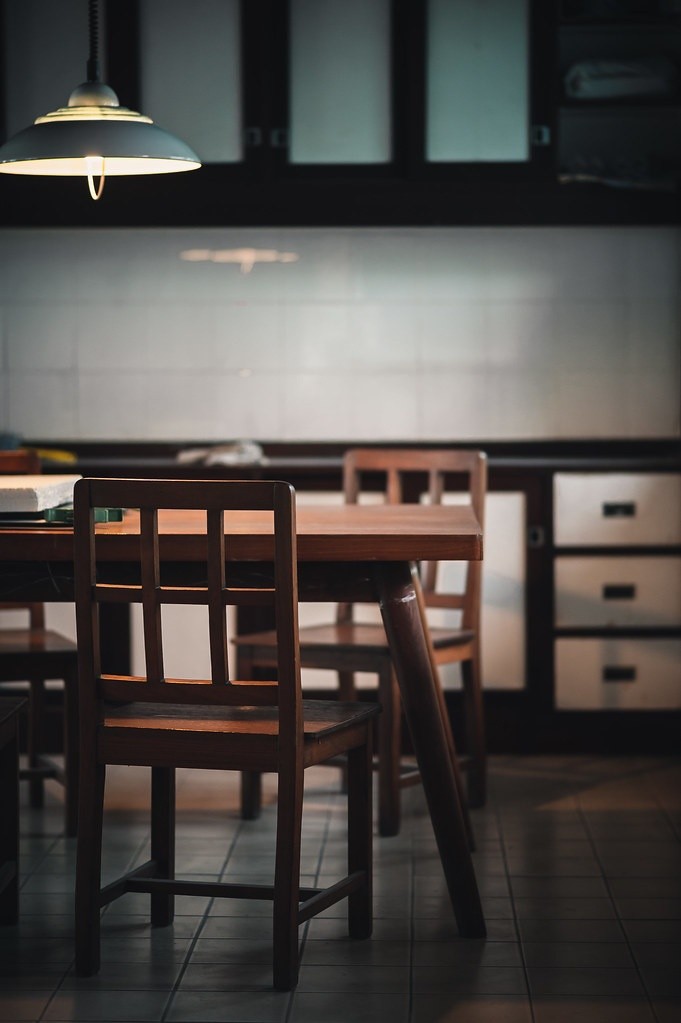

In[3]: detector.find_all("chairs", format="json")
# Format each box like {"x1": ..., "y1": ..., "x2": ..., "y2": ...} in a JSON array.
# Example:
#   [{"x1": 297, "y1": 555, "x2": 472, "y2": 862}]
[
  {"x1": 231, "y1": 450, "x2": 490, "y2": 837},
  {"x1": 72, "y1": 478, "x2": 381, "y2": 995},
  {"x1": 2, "y1": 449, "x2": 101, "y2": 851}
]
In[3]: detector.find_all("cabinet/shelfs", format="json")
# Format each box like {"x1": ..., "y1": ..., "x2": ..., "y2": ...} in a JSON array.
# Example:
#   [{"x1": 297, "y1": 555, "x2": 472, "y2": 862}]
[{"x1": 544, "y1": 459, "x2": 679, "y2": 755}]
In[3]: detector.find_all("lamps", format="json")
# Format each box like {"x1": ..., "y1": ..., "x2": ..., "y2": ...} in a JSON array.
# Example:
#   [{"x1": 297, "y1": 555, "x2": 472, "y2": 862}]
[{"x1": 1, "y1": 1, "x2": 201, "y2": 200}]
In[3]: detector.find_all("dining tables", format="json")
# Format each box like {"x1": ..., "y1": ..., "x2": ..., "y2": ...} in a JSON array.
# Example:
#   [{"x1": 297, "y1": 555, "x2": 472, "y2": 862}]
[{"x1": 0, "y1": 503, "x2": 486, "y2": 955}]
